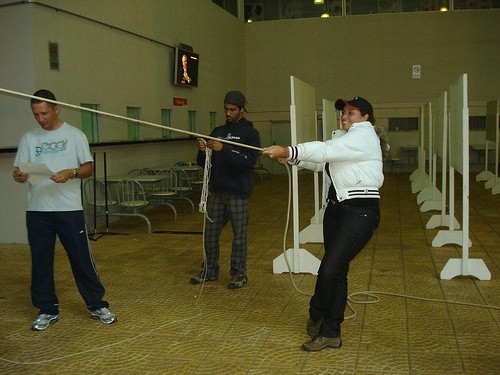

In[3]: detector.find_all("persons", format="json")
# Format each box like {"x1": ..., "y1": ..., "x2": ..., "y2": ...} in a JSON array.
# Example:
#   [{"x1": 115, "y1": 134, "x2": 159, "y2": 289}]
[
  {"x1": 189, "y1": 90, "x2": 261, "y2": 289},
  {"x1": 178, "y1": 54, "x2": 196, "y2": 85},
  {"x1": 12, "y1": 89, "x2": 118, "y2": 331},
  {"x1": 261, "y1": 96, "x2": 385, "y2": 352}
]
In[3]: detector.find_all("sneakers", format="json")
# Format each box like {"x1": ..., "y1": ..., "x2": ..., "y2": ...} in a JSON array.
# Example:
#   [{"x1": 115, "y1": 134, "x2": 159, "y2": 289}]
[
  {"x1": 89, "y1": 307, "x2": 117, "y2": 324},
  {"x1": 190, "y1": 270, "x2": 217, "y2": 283},
  {"x1": 32, "y1": 312, "x2": 61, "y2": 331},
  {"x1": 229, "y1": 274, "x2": 247, "y2": 289},
  {"x1": 302, "y1": 335, "x2": 342, "y2": 351},
  {"x1": 306, "y1": 317, "x2": 322, "y2": 336}
]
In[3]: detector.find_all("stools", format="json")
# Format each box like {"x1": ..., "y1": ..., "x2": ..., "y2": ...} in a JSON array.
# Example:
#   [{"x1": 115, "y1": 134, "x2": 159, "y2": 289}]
[
  {"x1": 84, "y1": 162, "x2": 202, "y2": 232},
  {"x1": 391, "y1": 155, "x2": 433, "y2": 177}
]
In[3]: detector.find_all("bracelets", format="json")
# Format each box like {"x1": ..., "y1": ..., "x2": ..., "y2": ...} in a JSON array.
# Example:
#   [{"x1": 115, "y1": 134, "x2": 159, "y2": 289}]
[{"x1": 72, "y1": 169, "x2": 77, "y2": 178}]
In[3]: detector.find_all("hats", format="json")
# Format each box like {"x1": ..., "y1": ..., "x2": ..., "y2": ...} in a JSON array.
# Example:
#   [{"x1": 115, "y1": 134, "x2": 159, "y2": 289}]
[
  {"x1": 334, "y1": 96, "x2": 373, "y2": 115},
  {"x1": 224, "y1": 91, "x2": 248, "y2": 113}
]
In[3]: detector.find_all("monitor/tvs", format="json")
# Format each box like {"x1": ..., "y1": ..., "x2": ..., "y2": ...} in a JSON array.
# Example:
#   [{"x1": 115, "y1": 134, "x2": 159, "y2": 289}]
[{"x1": 175, "y1": 46, "x2": 200, "y2": 88}]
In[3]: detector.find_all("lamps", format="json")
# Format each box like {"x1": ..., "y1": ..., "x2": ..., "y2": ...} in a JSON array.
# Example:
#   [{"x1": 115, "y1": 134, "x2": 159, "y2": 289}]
[
  {"x1": 321, "y1": 12, "x2": 329, "y2": 17},
  {"x1": 314, "y1": 0, "x2": 324, "y2": 5},
  {"x1": 439, "y1": 3, "x2": 449, "y2": 11}
]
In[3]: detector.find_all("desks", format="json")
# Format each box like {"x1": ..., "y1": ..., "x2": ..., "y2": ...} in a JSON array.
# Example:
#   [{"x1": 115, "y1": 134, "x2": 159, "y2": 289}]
[
  {"x1": 91, "y1": 175, "x2": 177, "y2": 233},
  {"x1": 149, "y1": 165, "x2": 202, "y2": 215},
  {"x1": 472, "y1": 144, "x2": 495, "y2": 160},
  {"x1": 401, "y1": 146, "x2": 418, "y2": 163},
  {"x1": 252, "y1": 151, "x2": 271, "y2": 181}
]
